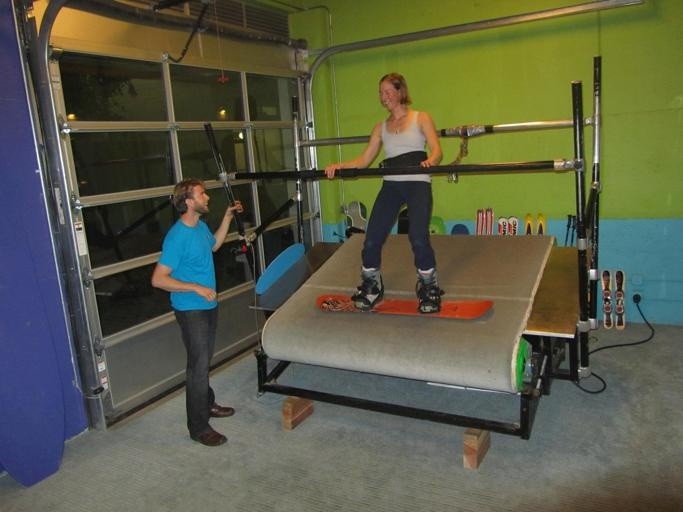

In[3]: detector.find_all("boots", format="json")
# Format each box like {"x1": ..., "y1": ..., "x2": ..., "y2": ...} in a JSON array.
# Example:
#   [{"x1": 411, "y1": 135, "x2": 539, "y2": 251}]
[
  {"x1": 415, "y1": 267, "x2": 446, "y2": 314},
  {"x1": 351, "y1": 265, "x2": 384, "y2": 312}
]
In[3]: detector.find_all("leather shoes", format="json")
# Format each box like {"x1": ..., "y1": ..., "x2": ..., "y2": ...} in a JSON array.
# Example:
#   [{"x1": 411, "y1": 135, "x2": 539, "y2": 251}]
[
  {"x1": 208, "y1": 401, "x2": 235, "y2": 418},
  {"x1": 190, "y1": 430, "x2": 228, "y2": 447}
]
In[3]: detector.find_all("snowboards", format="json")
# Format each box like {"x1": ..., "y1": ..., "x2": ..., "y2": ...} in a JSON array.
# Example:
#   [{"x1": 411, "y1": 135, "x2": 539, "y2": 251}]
[
  {"x1": 344, "y1": 200, "x2": 366, "y2": 239},
  {"x1": 398, "y1": 208, "x2": 410, "y2": 235},
  {"x1": 429, "y1": 216, "x2": 469, "y2": 235},
  {"x1": 317, "y1": 292, "x2": 493, "y2": 320}
]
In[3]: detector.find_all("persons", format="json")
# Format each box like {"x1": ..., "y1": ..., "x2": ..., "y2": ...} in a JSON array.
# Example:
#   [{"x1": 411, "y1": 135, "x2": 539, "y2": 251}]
[
  {"x1": 150, "y1": 179, "x2": 243, "y2": 446},
  {"x1": 327, "y1": 72, "x2": 442, "y2": 314}
]
